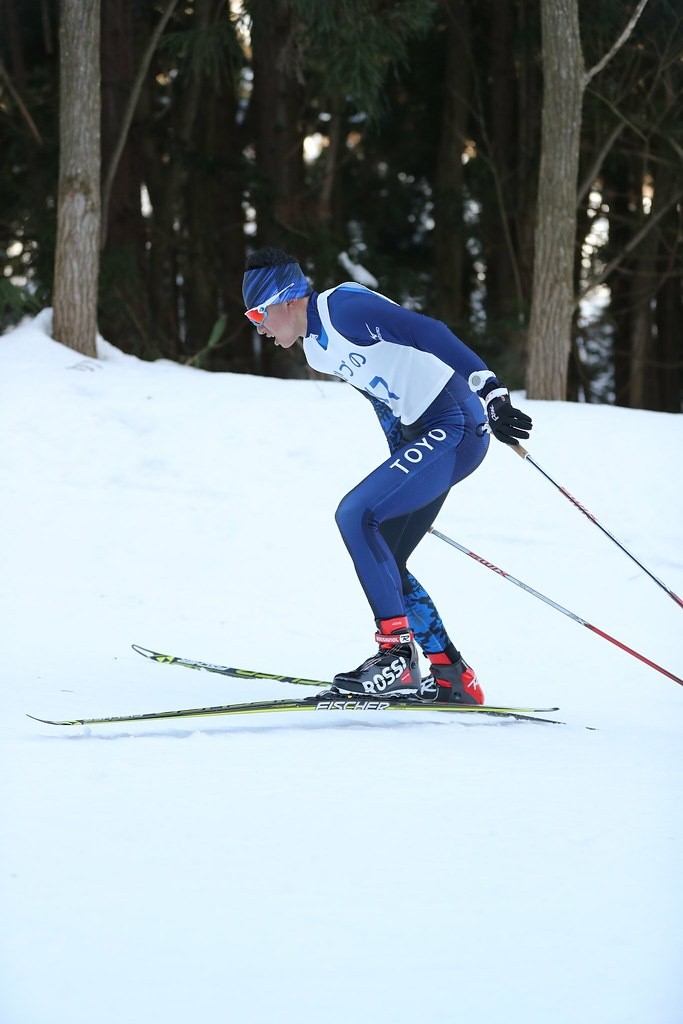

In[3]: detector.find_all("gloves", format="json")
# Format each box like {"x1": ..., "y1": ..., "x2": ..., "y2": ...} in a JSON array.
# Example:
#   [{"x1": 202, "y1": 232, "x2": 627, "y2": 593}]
[{"x1": 481, "y1": 385, "x2": 533, "y2": 447}]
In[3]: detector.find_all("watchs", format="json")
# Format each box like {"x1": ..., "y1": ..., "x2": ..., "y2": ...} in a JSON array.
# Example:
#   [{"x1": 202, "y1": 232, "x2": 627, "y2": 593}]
[{"x1": 469, "y1": 370, "x2": 495, "y2": 392}]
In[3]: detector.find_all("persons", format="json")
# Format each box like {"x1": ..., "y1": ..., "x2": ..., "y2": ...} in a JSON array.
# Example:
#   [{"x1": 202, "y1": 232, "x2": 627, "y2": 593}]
[{"x1": 242, "y1": 250, "x2": 532, "y2": 704}]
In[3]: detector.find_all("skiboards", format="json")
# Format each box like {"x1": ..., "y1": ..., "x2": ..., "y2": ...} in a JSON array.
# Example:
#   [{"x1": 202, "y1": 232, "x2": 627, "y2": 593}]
[{"x1": 22, "y1": 642, "x2": 599, "y2": 734}]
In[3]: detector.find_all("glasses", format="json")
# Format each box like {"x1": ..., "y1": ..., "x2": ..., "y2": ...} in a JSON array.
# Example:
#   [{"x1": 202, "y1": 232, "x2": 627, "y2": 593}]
[{"x1": 239, "y1": 283, "x2": 296, "y2": 327}]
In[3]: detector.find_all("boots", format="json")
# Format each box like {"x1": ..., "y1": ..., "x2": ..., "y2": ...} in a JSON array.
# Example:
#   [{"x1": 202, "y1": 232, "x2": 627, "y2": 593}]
[
  {"x1": 408, "y1": 643, "x2": 485, "y2": 705},
  {"x1": 333, "y1": 615, "x2": 422, "y2": 696}
]
